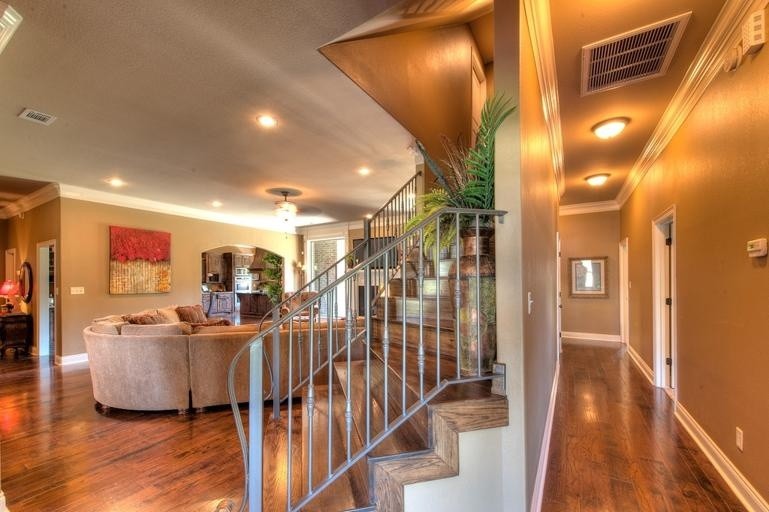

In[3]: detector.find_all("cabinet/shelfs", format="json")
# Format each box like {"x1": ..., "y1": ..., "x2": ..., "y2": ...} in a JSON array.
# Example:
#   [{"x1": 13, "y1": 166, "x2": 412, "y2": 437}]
[
  {"x1": 239, "y1": 294, "x2": 271, "y2": 320},
  {"x1": 203, "y1": 295, "x2": 234, "y2": 313}
]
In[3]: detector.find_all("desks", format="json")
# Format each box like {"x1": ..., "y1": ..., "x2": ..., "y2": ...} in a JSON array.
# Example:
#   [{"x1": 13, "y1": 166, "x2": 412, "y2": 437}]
[{"x1": 0, "y1": 313, "x2": 34, "y2": 357}]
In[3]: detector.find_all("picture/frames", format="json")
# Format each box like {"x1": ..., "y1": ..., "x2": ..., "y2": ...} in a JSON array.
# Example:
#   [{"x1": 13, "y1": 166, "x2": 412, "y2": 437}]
[{"x1": 568, "y1": 255, "x2": 609, "y2": 299}]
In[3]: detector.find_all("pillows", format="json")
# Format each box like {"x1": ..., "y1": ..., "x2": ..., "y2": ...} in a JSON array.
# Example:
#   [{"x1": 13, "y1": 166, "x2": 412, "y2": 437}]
[{"x1": 91, "y1": 305, "x2": 365, "y2": 335}]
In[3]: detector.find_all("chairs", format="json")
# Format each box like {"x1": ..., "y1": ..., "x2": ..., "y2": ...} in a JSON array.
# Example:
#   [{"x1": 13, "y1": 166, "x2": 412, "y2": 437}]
[{"x1": 281, "y1": 291, "x2": 319, "y2": 323}]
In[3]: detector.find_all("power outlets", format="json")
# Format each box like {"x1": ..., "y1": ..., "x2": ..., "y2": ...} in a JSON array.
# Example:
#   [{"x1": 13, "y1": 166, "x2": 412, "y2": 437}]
[{"x1": 735, "y1": 427, "x2": 743, "y2": 451}]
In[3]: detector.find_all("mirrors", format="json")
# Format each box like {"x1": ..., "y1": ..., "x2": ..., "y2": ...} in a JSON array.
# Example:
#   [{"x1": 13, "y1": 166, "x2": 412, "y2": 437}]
[{"x1": 18, "y1": 262, "x2": 33, "y2": 304}]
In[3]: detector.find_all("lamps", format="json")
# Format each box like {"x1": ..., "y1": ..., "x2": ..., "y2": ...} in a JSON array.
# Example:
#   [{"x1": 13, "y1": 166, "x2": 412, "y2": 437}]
[
  {"x1": 584, "y1": 173, "x2": 611, "y2": 184},
  {"x1": 591, "y1": 117, "x2": 631, "y2": 139},
  {"x1": 0, "y1": 280, "x2": 16, "y2": 312},
  {"x1": 273, "y1": 191, "x2": 297, "y2": 222}
]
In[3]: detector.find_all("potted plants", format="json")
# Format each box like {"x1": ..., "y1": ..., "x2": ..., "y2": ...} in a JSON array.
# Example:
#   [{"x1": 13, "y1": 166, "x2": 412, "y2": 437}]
[
  {"x1": 405, "y1": 90, "x2": 515, "y2": 376},
  {"x1": 345, "y1": 252, "x2": 353, "y2": 268}
]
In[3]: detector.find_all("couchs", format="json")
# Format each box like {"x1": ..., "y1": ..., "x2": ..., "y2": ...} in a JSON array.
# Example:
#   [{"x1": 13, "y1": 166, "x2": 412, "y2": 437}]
[{"x1": 84, "y1": 305, "x2": 365, "y2": 415}]
